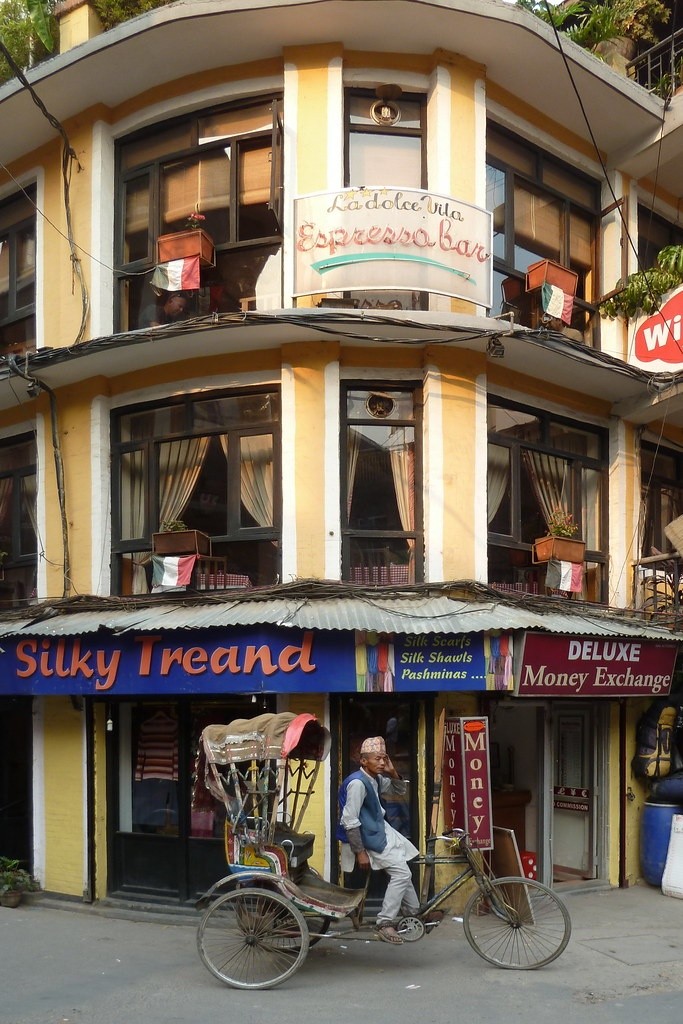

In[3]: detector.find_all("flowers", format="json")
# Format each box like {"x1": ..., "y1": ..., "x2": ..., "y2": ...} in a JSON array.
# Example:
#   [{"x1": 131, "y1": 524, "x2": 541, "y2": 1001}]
[
  {"x1": 545, "y1": 506, "x2": 580, "y2": 538},
  {"x1": 186, "y1": 213, "x2": 206, "y2": 229}
]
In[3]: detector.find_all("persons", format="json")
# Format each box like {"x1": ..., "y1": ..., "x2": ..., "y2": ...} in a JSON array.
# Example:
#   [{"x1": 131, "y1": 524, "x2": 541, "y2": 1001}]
[{"x1": 337, "y1": 736, "x2": 450, "y2": 946}]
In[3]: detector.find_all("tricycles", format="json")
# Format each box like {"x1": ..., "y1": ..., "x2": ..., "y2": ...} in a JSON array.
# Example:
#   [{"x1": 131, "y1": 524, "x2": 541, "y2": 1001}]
[{"x1": 194, "y1": 711, "x2": 571, "y2": 991}]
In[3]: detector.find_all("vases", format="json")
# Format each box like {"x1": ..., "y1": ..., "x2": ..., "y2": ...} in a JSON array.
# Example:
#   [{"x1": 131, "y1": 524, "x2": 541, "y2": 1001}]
[
  {"x1": 158, "y1": 228, "x2": 213, "y2": 266},
  {"x1": 535, "y1": 536, "x2": 584, "y2": 564}
]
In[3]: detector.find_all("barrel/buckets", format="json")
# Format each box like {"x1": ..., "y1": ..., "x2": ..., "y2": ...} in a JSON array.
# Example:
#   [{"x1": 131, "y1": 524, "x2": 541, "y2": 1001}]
[{"x1": 640, "y1": 801, "x2": 682, "y2": 888}]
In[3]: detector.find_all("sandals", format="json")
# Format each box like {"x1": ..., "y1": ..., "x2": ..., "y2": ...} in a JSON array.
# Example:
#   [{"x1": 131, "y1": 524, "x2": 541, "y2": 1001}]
[
  {"x1": 424, "y1": 907, "x2": 452, "y2": 934},
  {"x1": 373, "y1": 919, "x2": 403, "y2": 944}
]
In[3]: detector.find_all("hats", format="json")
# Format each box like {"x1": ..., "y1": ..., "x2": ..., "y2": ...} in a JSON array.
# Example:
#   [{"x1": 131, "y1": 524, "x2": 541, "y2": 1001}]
[{"x1": 361, "y1": 736, "x2": 386, "y2": 753}]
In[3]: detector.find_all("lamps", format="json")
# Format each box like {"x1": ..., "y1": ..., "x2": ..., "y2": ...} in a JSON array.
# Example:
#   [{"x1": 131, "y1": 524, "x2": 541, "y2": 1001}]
[{"x1": 372, "y1": 84, "x2": 402, "y2": 122}]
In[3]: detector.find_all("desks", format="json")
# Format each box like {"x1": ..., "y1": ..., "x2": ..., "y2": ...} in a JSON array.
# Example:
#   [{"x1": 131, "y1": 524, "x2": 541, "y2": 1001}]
[
  {"x1": 162, "y1": 574, "x2": 249, "y2": 592},
  {"x1": 350, "y1": 566, "x2": 409, "y2": 585},
  {"x1": 492, "y1": 788, "x2": 531, "y2": 850}
]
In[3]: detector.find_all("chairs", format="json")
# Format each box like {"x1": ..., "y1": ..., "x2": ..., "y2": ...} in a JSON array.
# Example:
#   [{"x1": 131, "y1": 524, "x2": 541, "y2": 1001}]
[{"x1": 230, "y1": 797, "x2": 315, "y2": 882}]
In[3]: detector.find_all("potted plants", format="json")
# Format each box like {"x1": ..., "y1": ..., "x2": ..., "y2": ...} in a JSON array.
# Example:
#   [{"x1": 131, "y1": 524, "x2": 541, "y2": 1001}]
[
  {"x1": 0, "y1": 856, "x2": 40, "y2": 907},
  {"x1": 155, "y1": 519, "x2": 208, "y2": 554},
  {"x1": 571, "y1": 0, "x2": 671, "y2": 61},
  {"x1": 645, "y1": 56, "x2": 683, "y2": 99},
  {"x1": 527, "y1": 259, "x2": 578, "y2": 300}
]
